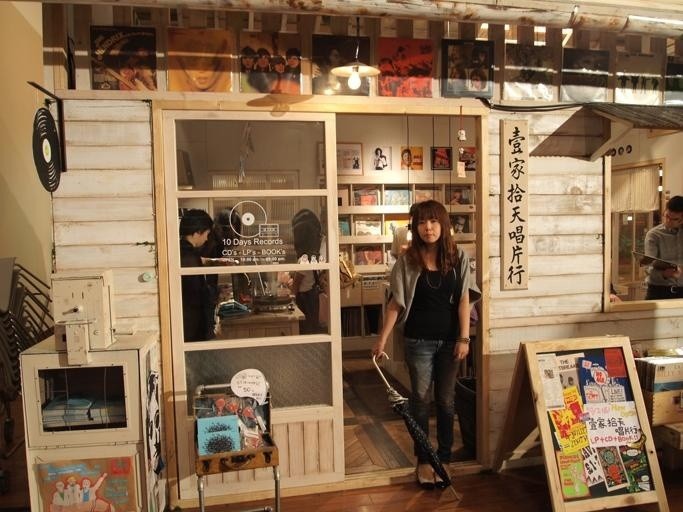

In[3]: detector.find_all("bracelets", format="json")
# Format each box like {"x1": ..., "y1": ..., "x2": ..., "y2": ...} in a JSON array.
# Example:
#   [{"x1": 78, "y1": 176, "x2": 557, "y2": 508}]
[{"x1": 455, "y1": 337, "x2": 471, "y2": 344}]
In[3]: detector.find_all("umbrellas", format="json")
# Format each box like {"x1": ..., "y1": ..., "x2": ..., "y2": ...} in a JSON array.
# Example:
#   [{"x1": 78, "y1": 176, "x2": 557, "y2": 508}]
[{"x1": 373, "y1": 352, "x2": 460, "y2": 501}]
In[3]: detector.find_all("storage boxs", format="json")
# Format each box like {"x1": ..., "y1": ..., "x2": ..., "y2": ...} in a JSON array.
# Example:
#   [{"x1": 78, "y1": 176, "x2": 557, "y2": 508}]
[{"x1": 638, "y1": 384, "x2": 682, "y2": 429}]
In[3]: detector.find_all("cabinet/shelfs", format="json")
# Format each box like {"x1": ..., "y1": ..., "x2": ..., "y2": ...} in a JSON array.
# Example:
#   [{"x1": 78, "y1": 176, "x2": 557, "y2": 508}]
[
  {"x1": 317, "y1": 170, "x2": 476, "y2": 280},
  {"x1": 340, "y1": 280, "x2": 386, "y2": 351},
  {"x1": 16, "y1": 328, "x2": 169, "y2": 512}
]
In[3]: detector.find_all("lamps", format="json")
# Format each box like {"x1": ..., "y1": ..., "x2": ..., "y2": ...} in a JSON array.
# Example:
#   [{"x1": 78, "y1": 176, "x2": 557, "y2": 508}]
[{"x1": 326, "y1": 16, "x2": 383, "y2": 92}]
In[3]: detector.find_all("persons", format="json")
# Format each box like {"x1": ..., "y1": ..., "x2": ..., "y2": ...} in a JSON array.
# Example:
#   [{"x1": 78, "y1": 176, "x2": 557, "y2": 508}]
[
  {"x1": 448, "y1": 45, "x2": 489, "y2": 92},
  {"x1": 401, "y1": 149, "x2": 414, "y2": 170},
  {"x1": 52, "y1": 472, "x2": 108, "y2": 512},
  {"x1": 644, "y1": 196, "x2": 683, "y2": 300},
  {"x1": 373, "y1": 148, "x2": 388, "y2": 170},
  {"x1": 118, "y1": 43, "x2": 157, "y2": 90},
  {"x1": 313, "y1": 46, "x2": 369, "y2": 96},
  {"x1": 372, "y1": 200, "x2": 482, "y2": 491},
  {"x1": 240, "y1": 46, "x2": 300, "y2": 95},
  {"x1": 170, "y1": 29, "x2": 230, "y2": 92},
  {"x1": 179, "y1": 209, "x2": 329, "y2": 343}
]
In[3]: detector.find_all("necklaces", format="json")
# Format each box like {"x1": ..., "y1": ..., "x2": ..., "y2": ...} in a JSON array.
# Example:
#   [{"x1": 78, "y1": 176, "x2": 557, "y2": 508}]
[{"x1": 426, "y1": 271, "x2": 442, "y2": 289}]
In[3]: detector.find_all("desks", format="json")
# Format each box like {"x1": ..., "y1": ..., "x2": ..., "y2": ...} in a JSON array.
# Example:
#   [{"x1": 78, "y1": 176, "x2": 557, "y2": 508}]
[
  {"x1": 212, "y1": 270, "x2": 308, "y2": 342},
  {"x1": 643, "y1": 418, "x2": 682, "y2": 487}
]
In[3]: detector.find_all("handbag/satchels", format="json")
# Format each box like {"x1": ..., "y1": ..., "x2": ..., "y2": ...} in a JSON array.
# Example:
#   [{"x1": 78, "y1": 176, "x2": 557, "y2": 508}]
[{"x1": 319, "y1": 260, "x2": 359, "y2": 292}]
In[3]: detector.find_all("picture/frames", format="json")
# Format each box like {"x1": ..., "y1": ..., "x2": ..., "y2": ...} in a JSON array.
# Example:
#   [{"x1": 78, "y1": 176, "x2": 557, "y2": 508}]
[
  {"x1": 428, "y1": 146, "x2": 453, "y2": 171},
  {"x1": 314, "y1": 141, "x2": 363, "y2": 178}
]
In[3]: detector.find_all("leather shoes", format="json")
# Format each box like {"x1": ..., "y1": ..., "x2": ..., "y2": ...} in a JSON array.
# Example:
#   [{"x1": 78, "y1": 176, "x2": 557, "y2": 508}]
[
  {"x1": 416, "y1": 471, "x2": 435, "y2": 490},
  {"x1": 434, "y1": 479, "x2": 446, "y2": 489}
]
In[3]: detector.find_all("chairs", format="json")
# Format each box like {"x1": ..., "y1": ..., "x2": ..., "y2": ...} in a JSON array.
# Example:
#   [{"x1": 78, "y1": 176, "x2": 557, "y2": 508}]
[
  {"x1": 191, "y1": 379, "x2": 281, "y2": 511},
  {"x1": 0, "y1": 250, "x2": 55, "y2": 468}
]
